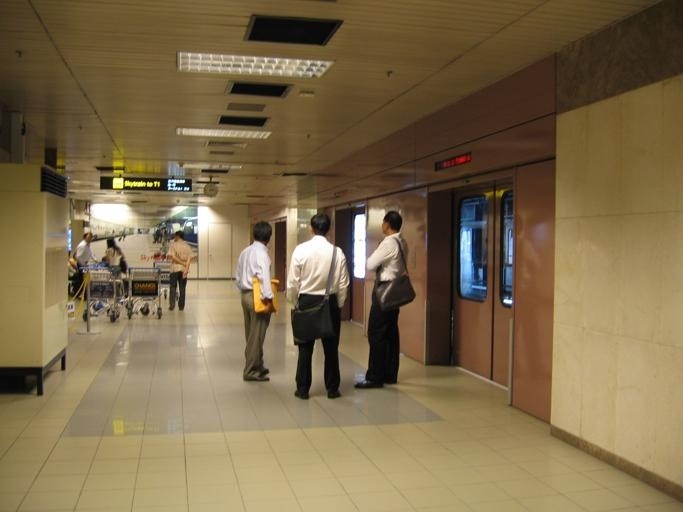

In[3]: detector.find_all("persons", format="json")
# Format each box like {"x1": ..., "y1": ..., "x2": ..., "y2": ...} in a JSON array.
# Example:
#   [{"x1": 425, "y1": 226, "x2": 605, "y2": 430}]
[
  {"x1": 76, "y1": 232, "x2": 98, "y2": 299},
  {"x1": 166, "y1": 231, "x2": 191, "y2": 310},
  {"x1": 285, "y1": 213, "x2": 350, "y2": 399},
  {"x1": 105, "y1": 238, "x2": 122, "y2": 274},
  {"x1": 235, "y1": 221, "x2": 274, "y2": 381},
  {"x1": 68, "y1": 250, "x2": 81, "y2": 292},
  {"x1": 354, "y1": 210, "x2": 408, "y2": 388}
]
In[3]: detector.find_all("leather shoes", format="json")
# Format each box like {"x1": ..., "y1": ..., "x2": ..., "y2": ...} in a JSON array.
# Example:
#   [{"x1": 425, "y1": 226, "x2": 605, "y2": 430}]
[
  {"x1": 294, "y1": 390, "x2": 309, "y2": 399},
  {"x1": 261, "y1": 369, "x2": 270, "y2": 375},
  {"x1": 382, "y1": 380, "x2": 398, "y2": 384},
  {"x1": 243, "y1": 373, "x2": 270, "y2": 382},
  {"x1": 328, "y1": 388, "x2": 342, "y2": 399},
  {"x1": 355, "y1": 380, "x2": 383, "y2": 390}
]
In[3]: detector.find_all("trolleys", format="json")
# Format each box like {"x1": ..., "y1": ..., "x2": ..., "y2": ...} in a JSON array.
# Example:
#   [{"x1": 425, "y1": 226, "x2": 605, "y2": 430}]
[{"x1": 83, "y1": 261, "x2": 180, "y2": 323}]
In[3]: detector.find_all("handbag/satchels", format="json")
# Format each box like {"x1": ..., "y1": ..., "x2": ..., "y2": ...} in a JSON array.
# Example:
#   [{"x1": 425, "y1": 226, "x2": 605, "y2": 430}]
[
  {"x1": 291, "y1": 299, "x2": 333, "y2": 345},
  {"x1": 373, "y1": 276, "x2": 416, "y2": 311}
]
